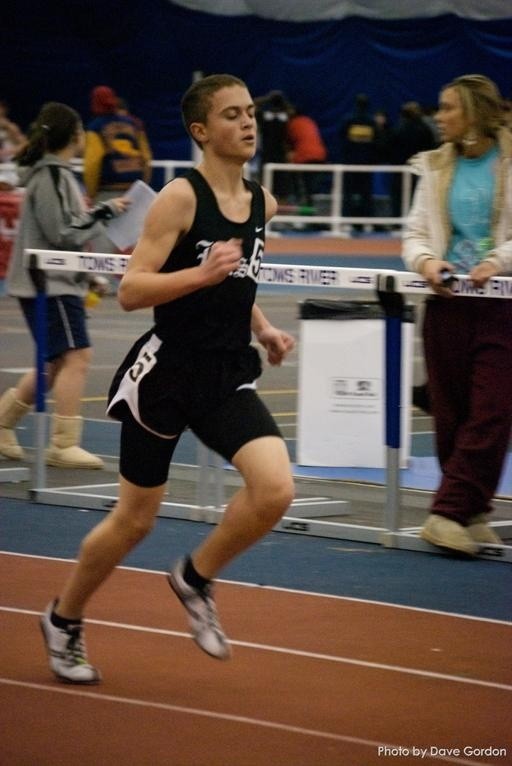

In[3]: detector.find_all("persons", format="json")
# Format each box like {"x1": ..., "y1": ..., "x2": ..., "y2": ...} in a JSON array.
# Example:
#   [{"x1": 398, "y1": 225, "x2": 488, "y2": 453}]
[
  {"x1": 0, "y1": 102, "x2": 132, "y2": 470},
  {"x1": 401, "y1": 75, "x2": 512, "y2": 554},
  {"x1": 41, "y1": 74, "x2": 297, "y2": 686},
  {"x1": 0, "y1": 87, "x2": 442, "y2": 308}
]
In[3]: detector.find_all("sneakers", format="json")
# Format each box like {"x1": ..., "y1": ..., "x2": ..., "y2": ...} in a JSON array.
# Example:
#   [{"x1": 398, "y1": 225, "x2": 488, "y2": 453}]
[
  {"x1": 172, "y1": 555, "x2": 228, "y2": 659},
  {"x1": 420, "y1": 514, "x2": 478, "y2": 555},
  {"x1": 38, "y1": 596, "x2": 101, "y2": 684},
  {"x1": 468, "y1": 513, "x2": 500, "y2": 544}
]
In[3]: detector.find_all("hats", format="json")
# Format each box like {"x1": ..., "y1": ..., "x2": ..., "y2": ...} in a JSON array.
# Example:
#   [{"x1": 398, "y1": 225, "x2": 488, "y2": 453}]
[{"x1": 93, "y1": 86, "x2": 117, "y2": 107}]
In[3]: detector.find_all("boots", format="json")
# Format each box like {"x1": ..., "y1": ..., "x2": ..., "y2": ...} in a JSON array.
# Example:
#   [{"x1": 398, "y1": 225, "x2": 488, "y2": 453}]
[
  {"x1": 46, "y1": 414, "x2": 104, "y2": 469},
  {"x1": 0, "y1": 388, "x2": 32, "y2": 459}
]
